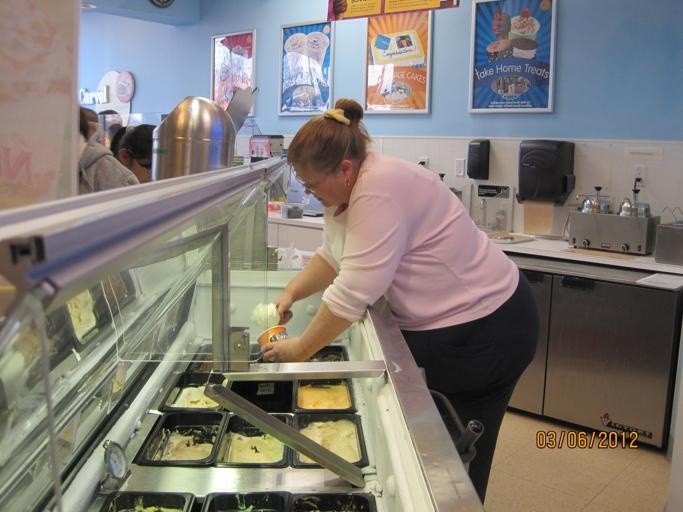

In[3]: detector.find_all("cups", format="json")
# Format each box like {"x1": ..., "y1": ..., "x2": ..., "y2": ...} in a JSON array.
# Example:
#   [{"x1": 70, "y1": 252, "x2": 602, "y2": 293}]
[
  {"x1": 508, "y1": 17, "x2": 541, "y2": 42},
  {"x1": 283, "y1": 32, "x2": 329, "y2": 80},
  {"x1": 258, "y1": 326, "x2": 288, "y2": 353}
]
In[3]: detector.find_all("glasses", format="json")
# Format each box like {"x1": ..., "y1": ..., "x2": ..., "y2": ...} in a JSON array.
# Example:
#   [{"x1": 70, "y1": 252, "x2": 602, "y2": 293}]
[{"x1": 294, "y1": 168, "x2": 335, "y2": 193}]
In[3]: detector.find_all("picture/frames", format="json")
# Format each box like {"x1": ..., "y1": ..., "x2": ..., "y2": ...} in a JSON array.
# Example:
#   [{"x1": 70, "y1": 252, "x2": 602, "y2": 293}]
[
  {"x1": 361, "y1": 9, "x2": 434, "y2": 116},
  {"x1": 275, "y1": 18, "x2": 335, "y2": 119},
  {"x1": 465, "y1": 1, "x2": 559, "y2": 118},
  {"x1": 208, "y1": 28, "x2": 256, "y2": 119}
]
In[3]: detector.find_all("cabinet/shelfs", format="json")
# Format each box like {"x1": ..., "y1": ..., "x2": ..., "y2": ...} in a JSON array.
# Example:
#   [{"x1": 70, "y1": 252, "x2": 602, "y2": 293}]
[{"x1": 506, "y1": 268, "x2": 682, "y2": 452}]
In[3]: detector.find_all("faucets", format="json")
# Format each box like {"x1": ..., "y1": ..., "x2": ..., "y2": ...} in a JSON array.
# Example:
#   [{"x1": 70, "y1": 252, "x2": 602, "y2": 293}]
[{"x1": 480, "y1": 197, "x2": 487, "y2": 228}]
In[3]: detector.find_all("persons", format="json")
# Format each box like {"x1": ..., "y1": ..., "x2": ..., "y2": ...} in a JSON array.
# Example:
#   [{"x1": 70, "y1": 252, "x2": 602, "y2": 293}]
[
  {"x1": 96, "y1": 108, "x2": 123, "y2": 151},
  {"x1": 79, "y1": 103, "x2": 139, "y2": 199},
  {"x1": 397, "y1": 35, "x2": 412, "y2": 49},
  {"x1": 261, "y1": 99, "x2": 539, "y2": 503},
  {"x1": 112, "y1": 125, "x2": 155, "y2": 180}
]
